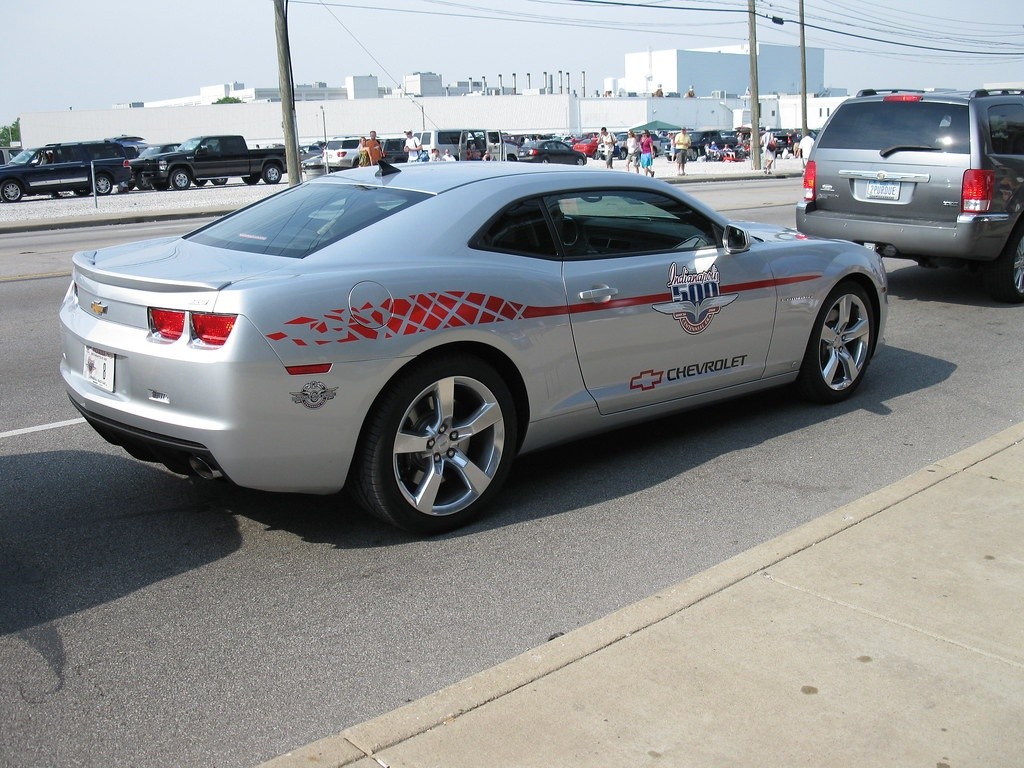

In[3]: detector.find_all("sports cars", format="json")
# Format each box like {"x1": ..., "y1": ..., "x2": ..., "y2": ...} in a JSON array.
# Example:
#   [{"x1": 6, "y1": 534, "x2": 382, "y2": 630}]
[{"x1": 55, "y1": 159, "x2": 889, "y2": 540}]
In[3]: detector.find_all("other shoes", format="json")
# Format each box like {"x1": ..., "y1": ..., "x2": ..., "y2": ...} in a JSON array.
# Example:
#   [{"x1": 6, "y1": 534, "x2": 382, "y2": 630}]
[
  {"x1": 768, "y1": 170, "x2": 772, "y2": 174},
  {"x1": 651, "y1": 171, "x2": 655, "y2": 178},
  {"x1": 678, "y1": 173, "x2": 689, "y2": 175},
  {"x1": 764, "y1": 166, "x2": 767, "y2": 174}
]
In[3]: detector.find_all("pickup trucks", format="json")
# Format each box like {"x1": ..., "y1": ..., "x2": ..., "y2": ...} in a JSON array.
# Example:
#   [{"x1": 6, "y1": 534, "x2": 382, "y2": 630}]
[{"x1": 143, "y1": 134, "x2": 287, "y2": 191}]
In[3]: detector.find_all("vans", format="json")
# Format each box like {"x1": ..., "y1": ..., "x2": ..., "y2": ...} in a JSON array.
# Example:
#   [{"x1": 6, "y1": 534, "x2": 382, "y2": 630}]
[{"x1": 413, "y1": 129, "x2": 520, "y2": 162}]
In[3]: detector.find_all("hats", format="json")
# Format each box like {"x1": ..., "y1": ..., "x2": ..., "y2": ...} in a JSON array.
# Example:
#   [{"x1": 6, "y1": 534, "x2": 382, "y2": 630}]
[
  {"x1": 766, "y1": 126, "x2": 770, "y2": 131},
  {"x1": 681, "y1": 127, "x2": 686, "y2": 130},
  {"x1": 403, "y1": 128, "x2": 412, "y2": 134}
]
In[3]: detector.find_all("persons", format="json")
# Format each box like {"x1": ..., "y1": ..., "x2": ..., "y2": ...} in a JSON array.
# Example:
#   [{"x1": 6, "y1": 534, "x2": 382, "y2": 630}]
[
  {"x1": 639, "y1": 128, "x2": 655, "y2": 177},
  {"x1": 430, "y1": 150, "x2": 442, "y2": 161},
  {"x1": 441, "y1": 149, "x2": 457, "y2": 161},
  {"x1": 675, "y1": 127, "x2": 691, "y2": 175},
  {"x1": 668, "y1": 132, "x2": 678, "y2": 162},
  {"x1": 625, "y1": 128, "x2": 640, "y2": 173},
  {"x1": 761, "y1": 126, "x2": 776, "y2": 175},
  {"x1": 704, "y1": 142, "x2": 733, "y2": 160},
  {"x1": 737, "y1": 131, "x2": 745, "y2": 147},
  {"x1": 404, "y1": 129, "x2": 423, "y2": 162},
  {"x1": 357, "y1": 137, "x2": 366, "y2": 167},
  {"x1": 482, "y1": 151, "x2": 494, "y2": 161},
  {"x1": 786, "y1": 129, "x2": 802, "y2": 158},
  {"x1": 596, "y1": 127, "x2": 618, "y2": 169},
  {"x1": 795, "y1": 129, "x2": 815, "y2": 165},
  {"x1": 367, "y1": 130, "x2": 386, "y2": 166}
]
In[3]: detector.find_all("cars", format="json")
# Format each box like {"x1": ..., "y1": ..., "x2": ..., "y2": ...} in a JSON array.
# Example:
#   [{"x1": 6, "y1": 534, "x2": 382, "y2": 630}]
[
  {"x1": 553, "y1": 130, "x2": 821, "y2": 162},
  {"x1": 99, "y1": 134, "x2": 182, "y2": 191},
  {"x1": 516, "y1": 139, "x2": 588, "y2": 166},
  {"x1": 288, "y1": 142, "x2": 326, "y2": 180},
  {"x1": 0, "y1": 146, "x2": 33, "y2": 166}
]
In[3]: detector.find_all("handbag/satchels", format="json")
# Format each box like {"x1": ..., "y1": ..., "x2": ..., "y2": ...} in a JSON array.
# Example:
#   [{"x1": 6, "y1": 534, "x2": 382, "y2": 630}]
[
  {"x1": 417, "y1": 151, "x2": 430, "y2": 162},
  {"x1": 766, "y1": 141, "x2": 777, "y2": 152}
]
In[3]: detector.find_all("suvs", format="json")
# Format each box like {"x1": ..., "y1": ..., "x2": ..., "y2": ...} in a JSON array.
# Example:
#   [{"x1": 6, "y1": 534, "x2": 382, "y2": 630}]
[
  {"x1": 0, "y1": 141, "x2": 133, "y2": 204},
  {"x1": 363, "y1": 138, "x2": 408, "y2": 163},
  {"x1": 795, "y1": 85, "x2": 1024, "y2": 303},
  {"x1": 321, "y1": 136, "x2": 380, "y2": 170}
]
